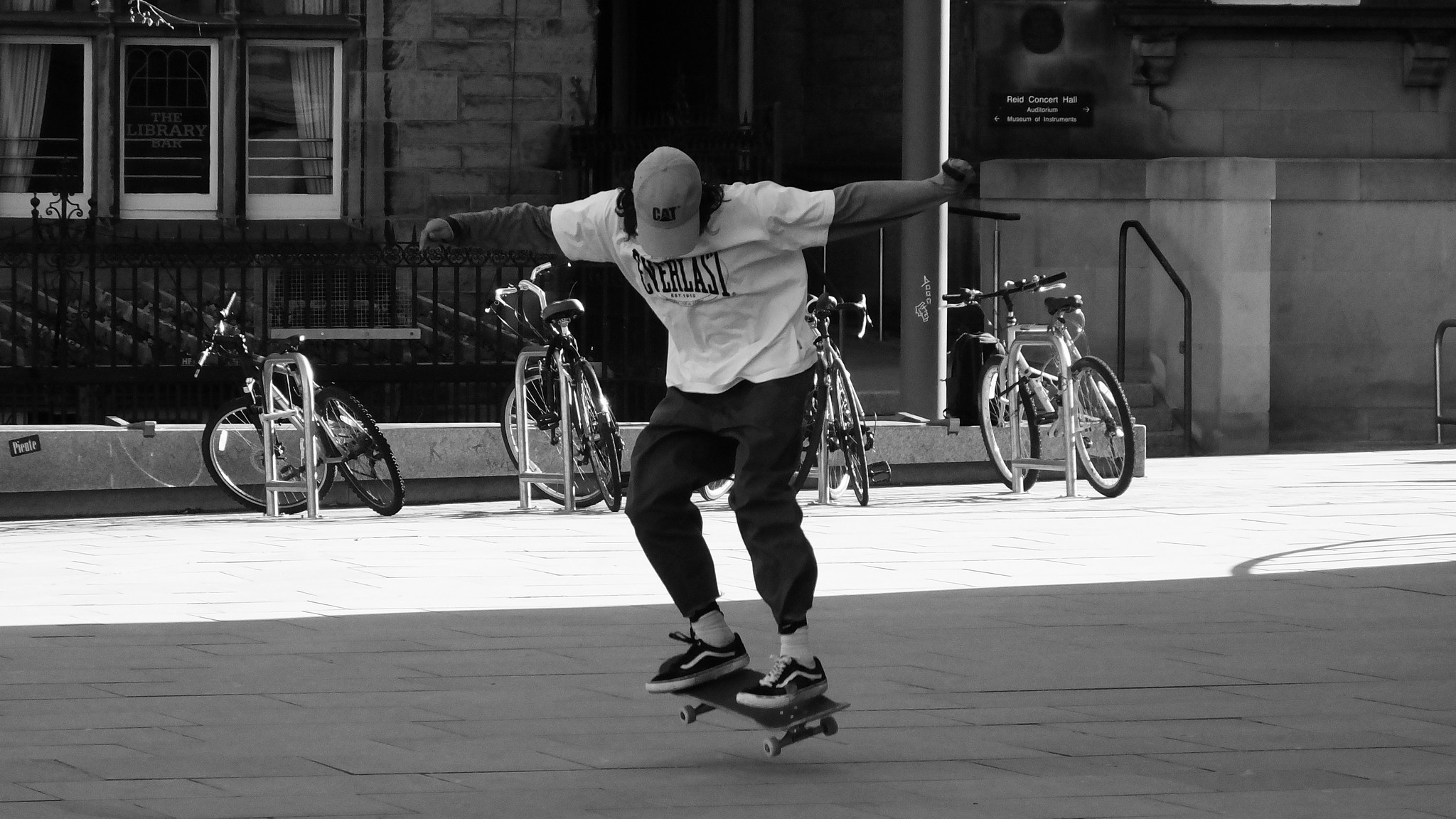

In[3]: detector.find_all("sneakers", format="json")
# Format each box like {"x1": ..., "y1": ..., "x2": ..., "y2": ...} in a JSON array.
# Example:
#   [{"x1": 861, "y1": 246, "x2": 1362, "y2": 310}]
[
  {"x1": 735, "y1": 654, "x2": 828, "y2": 709},
  {"x1": 644, "y1": 630, "x2": 750, "y2": 694}
]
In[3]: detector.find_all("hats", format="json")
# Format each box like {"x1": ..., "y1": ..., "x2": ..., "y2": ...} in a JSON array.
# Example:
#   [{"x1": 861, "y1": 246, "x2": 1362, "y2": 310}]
[{"x1": 632, "y1": 146, "x2": 703, "y2": 258}]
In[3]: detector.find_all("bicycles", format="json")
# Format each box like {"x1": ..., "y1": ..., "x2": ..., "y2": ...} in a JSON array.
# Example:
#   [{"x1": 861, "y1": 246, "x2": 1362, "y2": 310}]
[
  {"x1": 488, "y1": 260, "x2": 634, "y2": 514},
  {"x1": 941, "y1": 270, "x2": 1139, "y2": 499},
  {"x1": 190, "y1": 290, "x2": 409, "y2": 517},
  {"x1": 798, "y1": 289, "x2": 893, "y2": 506},
  {"x1": 697, "y1": 472, "x2": 735, "y2": 501}
]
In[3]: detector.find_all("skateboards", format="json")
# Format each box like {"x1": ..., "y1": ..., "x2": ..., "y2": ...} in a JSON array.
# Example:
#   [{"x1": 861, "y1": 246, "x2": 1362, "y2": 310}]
[{"x1": 656, "y1": 651, "x2": 849, "y2": 757}]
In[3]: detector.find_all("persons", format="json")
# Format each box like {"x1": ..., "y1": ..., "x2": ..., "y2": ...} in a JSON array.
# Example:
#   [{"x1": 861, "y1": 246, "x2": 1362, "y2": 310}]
[{"x1": 417, "y1": 144, "x2": 978, "y2": 705}]
